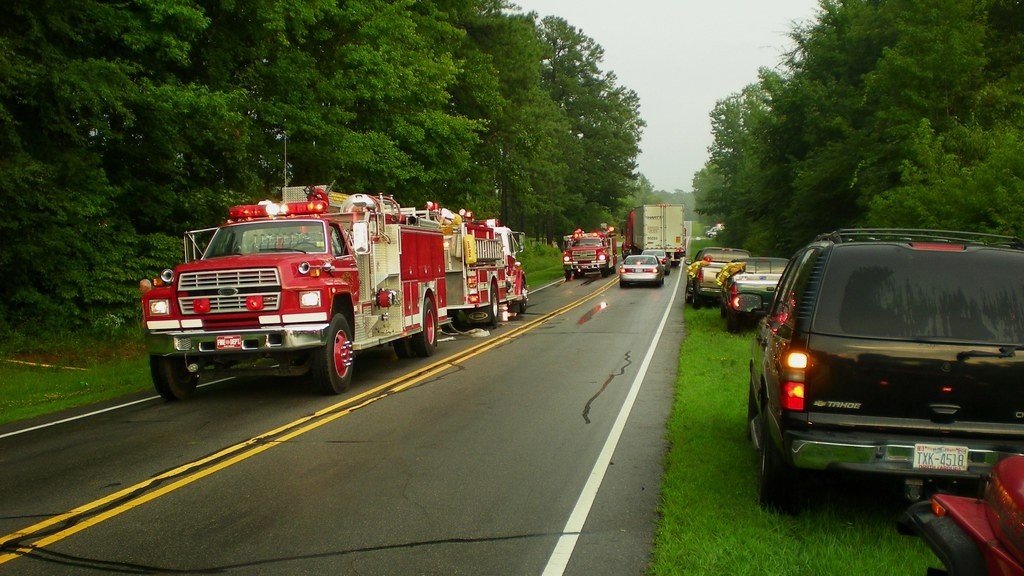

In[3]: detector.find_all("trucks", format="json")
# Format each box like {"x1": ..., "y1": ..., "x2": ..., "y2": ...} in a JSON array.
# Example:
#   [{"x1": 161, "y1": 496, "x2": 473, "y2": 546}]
[{"x1": 619, "y1": 204, "x2": 686, "y2": 267}]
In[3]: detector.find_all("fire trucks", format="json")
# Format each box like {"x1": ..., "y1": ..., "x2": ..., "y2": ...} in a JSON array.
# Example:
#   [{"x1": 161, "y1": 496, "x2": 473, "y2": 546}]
[
  {"x1": 140, "y1": 179, "x2": 453, "y2": 403},
  {"x1": 562, "y1": 223, "x2": 618, "y2": 280},
  {"x1": 439, "y1": 208, "x2": 529, "y2": 334}
]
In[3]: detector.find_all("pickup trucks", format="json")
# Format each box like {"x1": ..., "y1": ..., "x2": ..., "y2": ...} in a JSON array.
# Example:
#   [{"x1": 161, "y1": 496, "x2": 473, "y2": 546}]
[
  {"x1": 685, "y1": 247, "x2": 753, "y2": 309},
  {"x1": 715, "y1": 256, "x2": 791, "y2": 335}
]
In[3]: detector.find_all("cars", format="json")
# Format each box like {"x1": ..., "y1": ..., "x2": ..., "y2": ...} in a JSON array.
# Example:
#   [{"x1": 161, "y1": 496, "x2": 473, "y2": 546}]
[
  {"x1": 619, "y1": 254, "x2": 665, "y2": 288},
  {"x1": 640, "y1": 249, "x2": 672, "y2": 276},
  {"x1": 705, "y1": 227, "x2": 721, "y2": 238}
]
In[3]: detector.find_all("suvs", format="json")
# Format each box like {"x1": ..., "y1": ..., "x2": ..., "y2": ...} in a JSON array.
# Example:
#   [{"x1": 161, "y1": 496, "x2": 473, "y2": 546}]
[{"x1": 730, "y1": 228, "x2": 1024, "y2": 517}]
